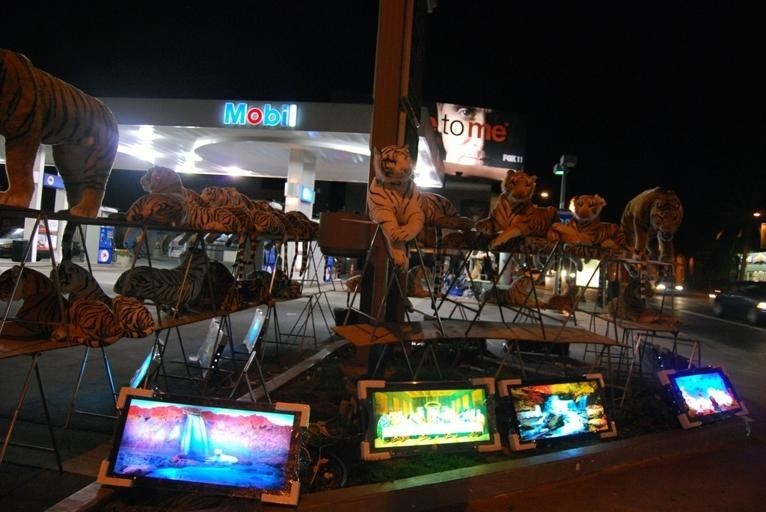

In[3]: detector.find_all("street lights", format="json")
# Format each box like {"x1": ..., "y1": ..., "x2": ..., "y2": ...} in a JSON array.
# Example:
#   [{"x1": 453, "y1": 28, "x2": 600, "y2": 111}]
[{"x1": 552, "y1": 153, "x2": 577, "y2": 294}]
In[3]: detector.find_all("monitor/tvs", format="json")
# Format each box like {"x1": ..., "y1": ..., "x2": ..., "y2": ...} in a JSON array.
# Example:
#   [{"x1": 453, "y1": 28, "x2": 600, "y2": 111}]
[
  {"x1": 95, "y1": 386, "x2": 311, "y2": 507},
  {"x1": 358, "y1": 377, "x2": 503, "y2": 460},
  {"x1": 497, "y1": 372, "x2": 619, "y2": 453},
  {"x1": 658, "y1": 364, "x2": 749, "y2": 431}
]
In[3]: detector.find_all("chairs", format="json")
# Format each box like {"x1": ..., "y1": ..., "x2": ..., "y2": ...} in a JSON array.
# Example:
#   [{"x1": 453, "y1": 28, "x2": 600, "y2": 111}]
[{"x1": 272, "y1": 233, "x2": 336, "y2": 374}]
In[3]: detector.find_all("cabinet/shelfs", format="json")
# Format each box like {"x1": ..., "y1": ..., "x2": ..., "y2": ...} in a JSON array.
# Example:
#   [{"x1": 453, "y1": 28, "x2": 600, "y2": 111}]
[
  {"x1": 319, "y1": 211, "x2": 701, "y2": 428},
  {"x1": 0, "y1": 205, "x2": 272, "y2": 474}
]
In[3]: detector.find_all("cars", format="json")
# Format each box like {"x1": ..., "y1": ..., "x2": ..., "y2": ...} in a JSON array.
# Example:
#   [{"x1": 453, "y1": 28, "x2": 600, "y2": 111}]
[
  {"x1": 640, "y1": 274, "x2": 685, "y2": 296},
  {"x1": 711, "y1": 280, "x2": 766, "y2": 326}
]
